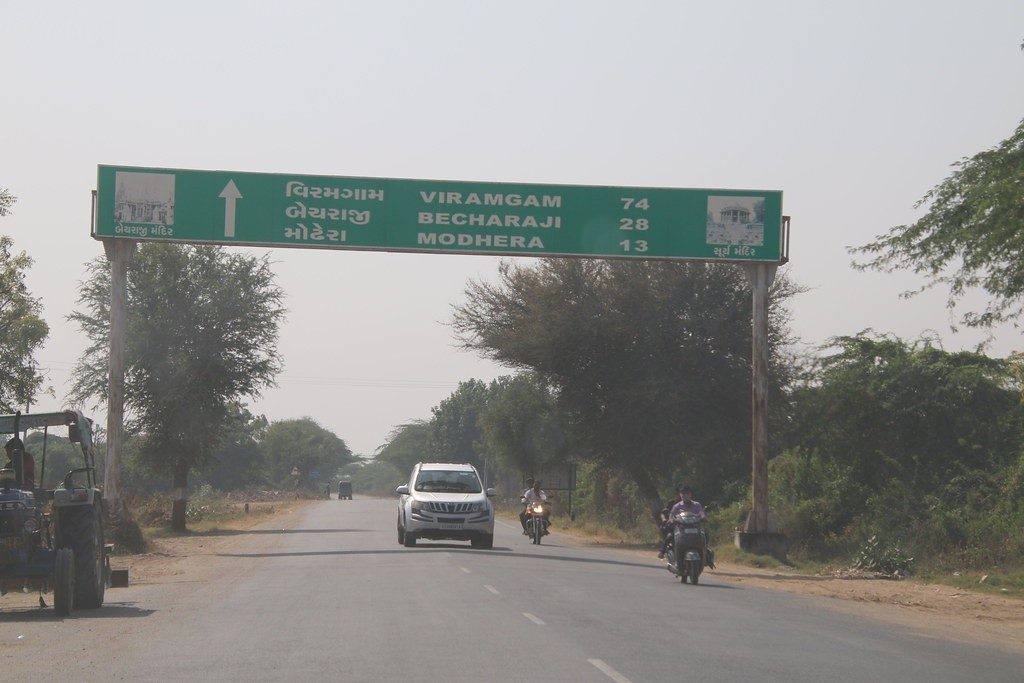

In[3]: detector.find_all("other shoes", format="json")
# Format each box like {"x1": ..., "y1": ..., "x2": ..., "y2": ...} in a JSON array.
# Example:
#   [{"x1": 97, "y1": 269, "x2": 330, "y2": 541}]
[
  {"x1": 658, "y1": 552, "x2": 664, "y2": 558},
  {"x1": 523, "y1": 529, "x2": 528, "y2": 535},
  {"x1": 544, "y1": 530, "x2": 550, "y2": 534}
]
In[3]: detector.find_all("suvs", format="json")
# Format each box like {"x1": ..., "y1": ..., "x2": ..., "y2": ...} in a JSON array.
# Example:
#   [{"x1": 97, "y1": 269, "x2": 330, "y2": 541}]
[{"x1": 395, "y1": 462, "x2": 497, "y2": 550}]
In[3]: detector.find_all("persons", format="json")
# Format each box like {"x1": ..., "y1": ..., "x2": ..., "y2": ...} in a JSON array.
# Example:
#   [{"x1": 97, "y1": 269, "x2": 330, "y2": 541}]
[
  {"x1": 657, "y1": 482, "x2": 714, "y2": 562},
  {"x1": 519, "y1": 478, "x2": 551, "y2": 536},
  {"x1": 3, "y1": 438, "x2": 35, "y2": 491}
]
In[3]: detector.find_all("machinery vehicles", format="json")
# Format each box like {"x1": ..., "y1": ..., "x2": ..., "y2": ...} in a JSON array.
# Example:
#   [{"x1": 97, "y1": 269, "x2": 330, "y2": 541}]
[{"x1": 0, "y1": 409, "x2": 128, "y2": 608}]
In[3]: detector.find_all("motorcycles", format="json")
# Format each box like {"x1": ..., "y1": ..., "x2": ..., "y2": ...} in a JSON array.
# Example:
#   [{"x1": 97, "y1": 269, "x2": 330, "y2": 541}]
[
  {"x1": 520, "y1": 493, "x2": 554, "y2": 544},
  {"x1": 662, "y1": 505, "x2": 714, "y2": 585}
]
